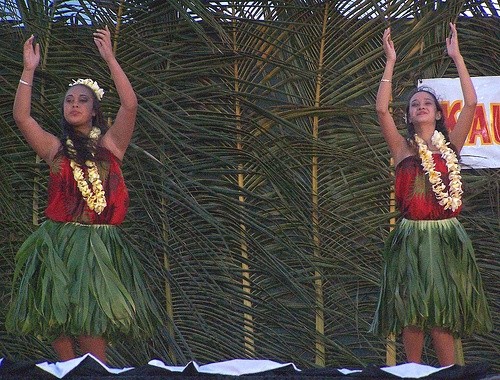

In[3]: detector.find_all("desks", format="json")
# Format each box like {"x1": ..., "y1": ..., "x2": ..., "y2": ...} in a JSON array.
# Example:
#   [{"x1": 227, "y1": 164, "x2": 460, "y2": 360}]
[{"x1": 0, "y1": 356, "x2": 500, "y2": 380}]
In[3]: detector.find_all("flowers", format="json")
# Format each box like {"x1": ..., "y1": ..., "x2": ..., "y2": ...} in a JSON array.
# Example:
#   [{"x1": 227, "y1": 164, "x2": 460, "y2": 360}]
[
  {"x1": 410, "y1": 128, "x2": 463, "y2": 212},
  {"x1": 61, "y1": 125, "x2": 108, "y2": 216}
]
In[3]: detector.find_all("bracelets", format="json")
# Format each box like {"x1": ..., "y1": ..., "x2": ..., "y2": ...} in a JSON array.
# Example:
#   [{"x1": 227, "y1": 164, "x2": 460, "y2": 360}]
[
  {"x1": 381, "y1": 79, "x2": 394, "y2": 85},
  {"x1": 19, "y1": 77, "x2": 34, "y2": 88}
]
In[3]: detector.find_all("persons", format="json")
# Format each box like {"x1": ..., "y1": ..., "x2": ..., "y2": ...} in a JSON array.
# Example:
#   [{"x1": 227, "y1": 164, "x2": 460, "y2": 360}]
[
  {"x1": 10, "y1": 23, "x2": 148, "y2": 364},
  {"x1": 374, "y1": 21, "x2": 481, "y2": 369}
]
